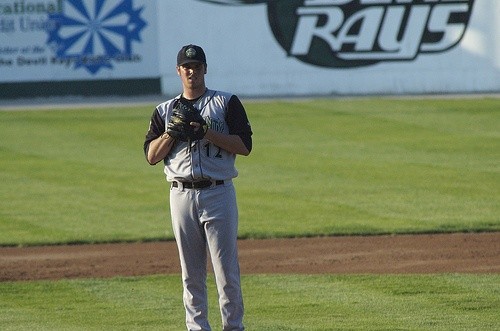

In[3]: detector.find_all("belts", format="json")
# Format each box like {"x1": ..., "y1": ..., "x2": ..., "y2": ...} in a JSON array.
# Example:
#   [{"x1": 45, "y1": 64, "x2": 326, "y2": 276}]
[{"x1": 173, "y1": 180, "x2": 224, "y2": 190}]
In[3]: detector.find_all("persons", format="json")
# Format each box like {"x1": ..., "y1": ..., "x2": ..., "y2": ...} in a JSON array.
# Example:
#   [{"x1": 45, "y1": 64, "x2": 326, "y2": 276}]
[{"x1": 144, "y1": 45, "x2": 253, "y2": 331}]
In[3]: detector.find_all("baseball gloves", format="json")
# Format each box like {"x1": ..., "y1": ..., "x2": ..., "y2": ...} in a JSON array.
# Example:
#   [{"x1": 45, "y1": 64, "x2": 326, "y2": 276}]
[{"x1": 167, "y1": 98, "x2": 207, "y2": 140}]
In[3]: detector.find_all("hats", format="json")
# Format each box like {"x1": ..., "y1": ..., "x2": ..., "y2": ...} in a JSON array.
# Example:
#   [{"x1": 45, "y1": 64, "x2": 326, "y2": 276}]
[{"x1": 177, "y1": 44, "x2": 206, "y2": 67}]
